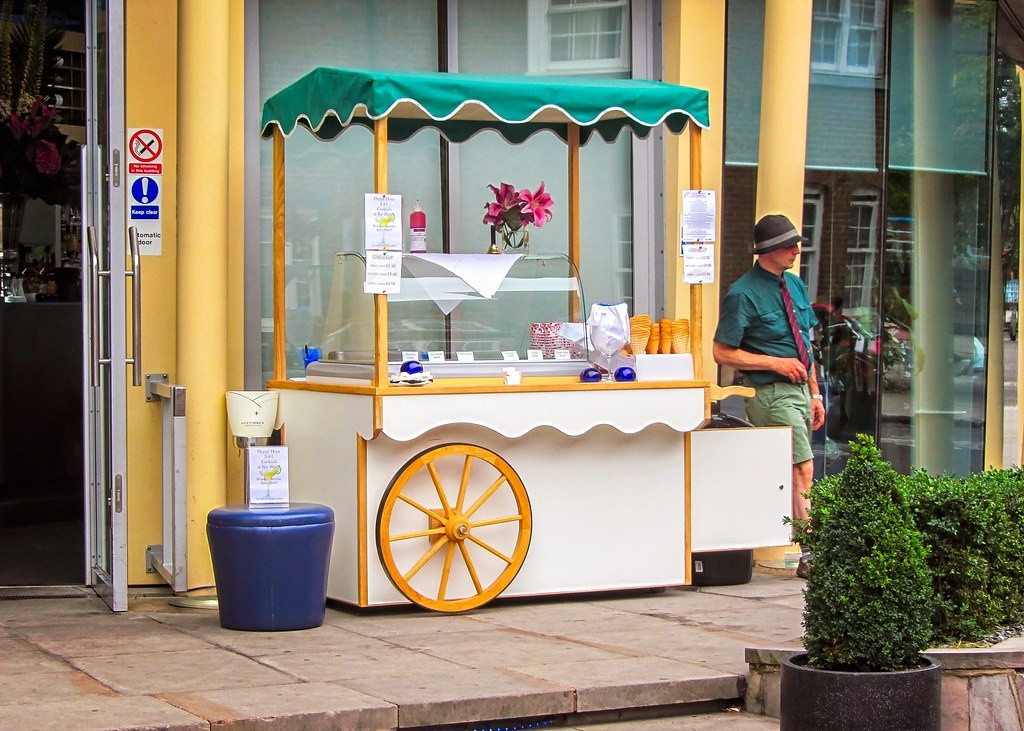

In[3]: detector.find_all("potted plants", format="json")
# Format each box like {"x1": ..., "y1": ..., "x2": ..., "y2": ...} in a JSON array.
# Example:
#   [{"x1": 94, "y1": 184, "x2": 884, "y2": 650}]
[{"x1": 781, "y1": 432, "x2": 941, "y2": 731}]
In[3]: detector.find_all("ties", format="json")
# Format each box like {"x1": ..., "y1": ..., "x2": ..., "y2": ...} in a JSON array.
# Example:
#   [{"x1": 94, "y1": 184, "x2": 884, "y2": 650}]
[{"x1": 779, "y1": 277, "x2": 811, "y2": 371}]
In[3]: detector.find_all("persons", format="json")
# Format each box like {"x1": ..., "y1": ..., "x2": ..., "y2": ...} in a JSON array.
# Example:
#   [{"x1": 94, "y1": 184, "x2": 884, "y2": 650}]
[{"x1": 712, "y1": 215, "x2": 825, "y2": 578}]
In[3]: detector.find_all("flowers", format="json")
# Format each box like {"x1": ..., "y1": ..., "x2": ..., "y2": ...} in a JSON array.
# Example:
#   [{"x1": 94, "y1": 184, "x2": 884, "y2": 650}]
[
  {"x1": 0, "y1": 99, "x2": 82, "y2": 194},
  {"x1": 483, "y1": 181, "x2": 554, "y2": 248}
]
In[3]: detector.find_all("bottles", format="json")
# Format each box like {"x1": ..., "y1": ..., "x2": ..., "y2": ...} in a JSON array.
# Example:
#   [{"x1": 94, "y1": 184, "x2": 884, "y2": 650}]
[{"x1": 410, "y1": 199, "x2": 427, "y2": 253}]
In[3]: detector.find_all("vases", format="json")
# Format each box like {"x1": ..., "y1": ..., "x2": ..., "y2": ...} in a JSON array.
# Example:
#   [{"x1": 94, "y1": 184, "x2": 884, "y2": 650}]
[
  {"x1": 2, "y1": 195, "x2": 25, "y2": 288},
  {"x1": 502, "y1": 231, "x2": 528, "y2": 255}
]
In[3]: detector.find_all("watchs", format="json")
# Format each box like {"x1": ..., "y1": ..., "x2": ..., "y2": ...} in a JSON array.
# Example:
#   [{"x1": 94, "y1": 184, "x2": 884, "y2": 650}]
[{"x1": 811, "y1": 395, "x2": 823, "y2": 401}]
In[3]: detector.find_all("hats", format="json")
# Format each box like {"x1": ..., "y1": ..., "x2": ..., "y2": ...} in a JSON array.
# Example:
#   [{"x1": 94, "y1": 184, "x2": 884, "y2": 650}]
[{"x1": 750, "y1": 215, "x2": 809, "y2": 256}]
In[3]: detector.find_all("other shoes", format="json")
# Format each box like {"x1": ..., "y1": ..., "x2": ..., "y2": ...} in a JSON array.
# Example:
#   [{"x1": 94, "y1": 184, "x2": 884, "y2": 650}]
[{"x1": 796, "y1": 557, "x2": 813, "y2": 578}]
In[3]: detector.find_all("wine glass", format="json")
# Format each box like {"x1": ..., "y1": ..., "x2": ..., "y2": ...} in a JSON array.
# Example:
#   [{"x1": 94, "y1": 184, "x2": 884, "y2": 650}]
[{"x1": 590, "y1": 311, "x2": 624, "y2": 382}]
